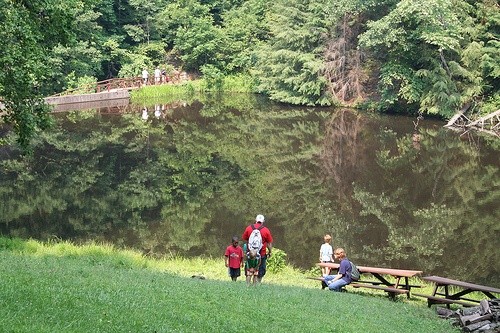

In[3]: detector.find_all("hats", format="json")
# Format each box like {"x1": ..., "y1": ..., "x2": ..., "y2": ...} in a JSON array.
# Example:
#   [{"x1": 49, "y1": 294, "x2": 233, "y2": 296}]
[{"x1": 256, "y1": 214, "x2": 264, "y2": 223}]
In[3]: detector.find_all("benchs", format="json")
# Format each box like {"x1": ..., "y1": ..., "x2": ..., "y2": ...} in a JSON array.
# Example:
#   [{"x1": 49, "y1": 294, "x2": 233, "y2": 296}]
[
  {"x1": 411, "y1": 293, "x2": 478, "y2": 309},
  {"x1": 316, "y1": 278, "x2": 408, "y2": 299},
  {"x1": 436, "y1": 293, "x2": 480, "y2": 302},
  {"x1": 358, "y1": 280, "x2": 422, "y2": 288}
]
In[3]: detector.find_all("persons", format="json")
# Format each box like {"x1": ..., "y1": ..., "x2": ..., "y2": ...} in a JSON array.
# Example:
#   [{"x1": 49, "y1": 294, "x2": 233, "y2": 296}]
[
  {"x1": 245, "y1": 249, "x2": 261, "y2": 287},
  {"x1": 142, "y1": 67, "x2": 150, "y2": 83},
  {"x1": 242, "y1": 214, "x2": 273, "y2": 283},
  {"x1": 142, "y1": 105, "x2": 169, "y2": 123},
  {"x1": 154, "y1": 66, "x2": 169, "y2": 85},
  {"x1": 324, "y1": 247, "x2": 352, "y2": 292},
  {"x1": 319, "y1": 234, "x2": 334, "y2": 277},
  {"x1": 224, "y1": 236, "x2": 244, "y2": 281}
]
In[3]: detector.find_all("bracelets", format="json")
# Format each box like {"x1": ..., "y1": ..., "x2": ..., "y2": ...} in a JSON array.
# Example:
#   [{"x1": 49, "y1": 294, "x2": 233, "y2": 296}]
[{"x1": 268, "y1": 252, "x2": 271, "y2": 255}]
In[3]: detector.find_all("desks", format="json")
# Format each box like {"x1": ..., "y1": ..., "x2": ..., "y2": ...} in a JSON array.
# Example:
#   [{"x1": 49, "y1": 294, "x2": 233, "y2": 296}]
[
  {"x1": 317, "y1": 263, "x2": 423, "y2": 299},
  {"x1": 423, "y1": 276, "x2": 500, "y2": 307}
]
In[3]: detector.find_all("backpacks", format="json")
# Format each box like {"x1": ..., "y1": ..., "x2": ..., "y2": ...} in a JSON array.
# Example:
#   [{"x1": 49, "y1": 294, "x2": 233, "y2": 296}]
[
  {"x1": 247, "y1": 224, "x2": 266, "y2": 253},
  {"x1": 345, "y1": 260, "x2": 360, "y2": 281}
]
